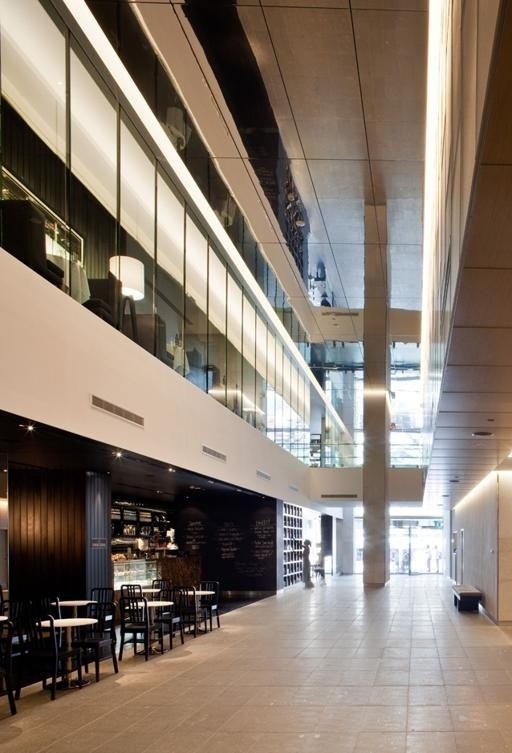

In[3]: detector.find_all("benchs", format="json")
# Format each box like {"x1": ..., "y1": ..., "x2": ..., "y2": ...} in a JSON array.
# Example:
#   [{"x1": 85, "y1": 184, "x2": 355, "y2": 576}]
[{"x1": 450, "y1": 584, "x2": 481, "y2": 613}]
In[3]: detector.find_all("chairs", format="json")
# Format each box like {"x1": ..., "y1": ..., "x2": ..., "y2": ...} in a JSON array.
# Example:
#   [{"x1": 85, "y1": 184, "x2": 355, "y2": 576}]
[
  {"x1": 1, "y1": 196, "x2": 141, "y2": 342},
  {"x1": 0, "y1": 577, "x2": 221, "y2": 715},
  {"x1": 122, "y1": 311, "x2": 218, "y2": 390}
]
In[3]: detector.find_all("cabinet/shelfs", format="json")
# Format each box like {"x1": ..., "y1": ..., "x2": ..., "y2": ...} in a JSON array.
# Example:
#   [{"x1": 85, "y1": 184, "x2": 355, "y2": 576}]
[{"x1": 283, "y1": 502, "x2": 303, "y2": 586}]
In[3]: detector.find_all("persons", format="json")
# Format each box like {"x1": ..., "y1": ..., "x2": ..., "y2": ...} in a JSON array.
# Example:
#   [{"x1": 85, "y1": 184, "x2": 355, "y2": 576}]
[{"x1": 292, "y1": 537, "x2": 316, "y2": 588}]
